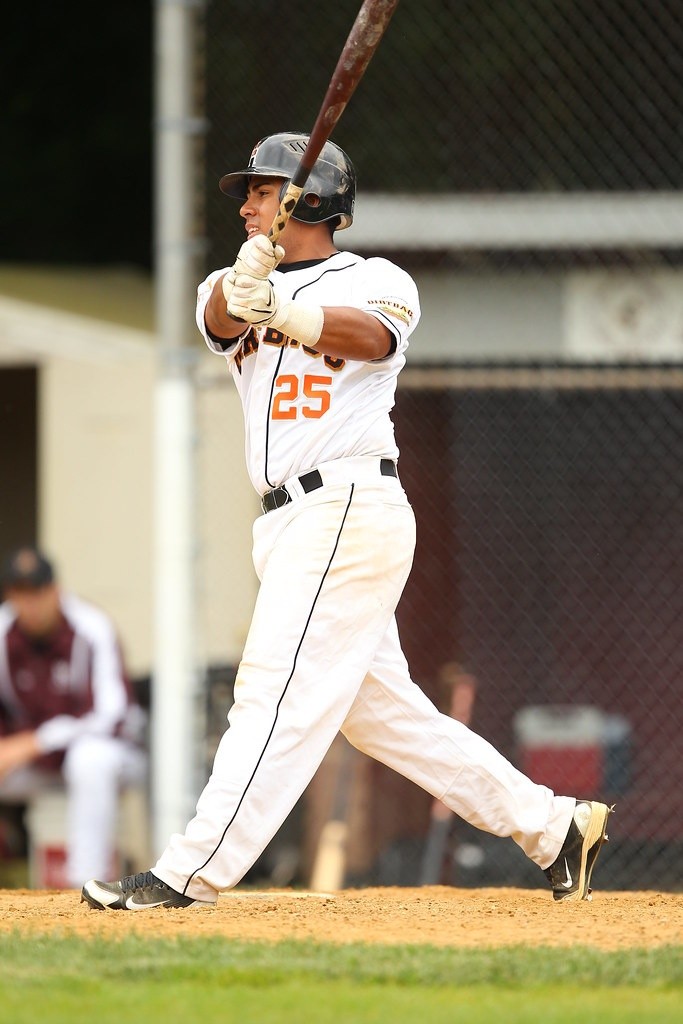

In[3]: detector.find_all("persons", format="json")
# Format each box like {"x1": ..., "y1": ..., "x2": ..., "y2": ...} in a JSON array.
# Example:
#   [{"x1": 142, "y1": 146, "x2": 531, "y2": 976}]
[
  {"x1": 1, "y1": 547, "x2": 148, "y2": 886},
  {"x1": 84, "y1": 133, "x2": 607, "y2": 911}
]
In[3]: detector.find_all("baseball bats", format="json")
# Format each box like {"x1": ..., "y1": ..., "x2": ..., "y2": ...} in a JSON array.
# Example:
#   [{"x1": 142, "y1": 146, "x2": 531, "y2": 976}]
[{"x1": 272, "y1": 0, "x2": 392, "y2": 245}]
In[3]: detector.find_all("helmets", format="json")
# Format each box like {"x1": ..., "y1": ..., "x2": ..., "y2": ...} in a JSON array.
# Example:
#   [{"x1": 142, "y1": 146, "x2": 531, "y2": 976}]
[{"x1": 218, "y1": 129, "x2": 358, "y2": 232}]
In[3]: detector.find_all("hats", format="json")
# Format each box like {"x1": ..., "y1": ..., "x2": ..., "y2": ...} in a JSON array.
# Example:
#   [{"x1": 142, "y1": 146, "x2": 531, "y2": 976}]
[{"x1": 0, "y1": 546, "x2": 53, "y2": 589}]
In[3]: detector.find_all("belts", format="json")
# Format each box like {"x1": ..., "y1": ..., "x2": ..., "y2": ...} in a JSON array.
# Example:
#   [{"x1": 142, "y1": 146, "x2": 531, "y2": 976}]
[{"x1": 260, "y1": 458, "x2": 396, "y2": 515}]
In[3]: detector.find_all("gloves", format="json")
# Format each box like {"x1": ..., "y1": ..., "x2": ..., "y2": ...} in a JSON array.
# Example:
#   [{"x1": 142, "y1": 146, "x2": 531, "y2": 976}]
[
  {"x1": 225, "y1": 274, "x2": 325, "y2": 348},
  {"x1": 221, "y1": 233, "x2": 286, "y2": 303}
]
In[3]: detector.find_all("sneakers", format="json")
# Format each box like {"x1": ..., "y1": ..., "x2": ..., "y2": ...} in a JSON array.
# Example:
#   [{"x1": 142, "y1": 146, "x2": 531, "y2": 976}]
[
  {"x1": 542, "y1": 797, "x2": 618, "y2": 903},
  {"x1": 79, "y1": 869, "x2": 216, "y2": 912}
]
